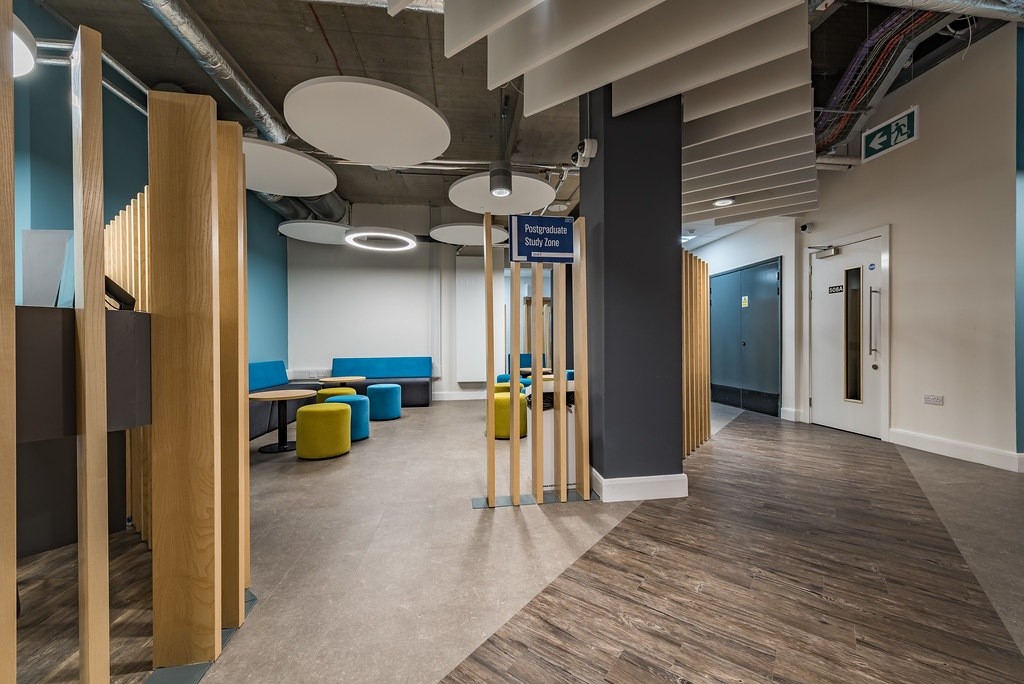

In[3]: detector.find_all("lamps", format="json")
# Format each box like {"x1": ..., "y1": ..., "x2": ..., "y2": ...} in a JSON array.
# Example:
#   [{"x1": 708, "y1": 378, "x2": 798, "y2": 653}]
[
  {"x1": 713, "y1": 199, "x2": 733, "y2": 208},
  {"x1": 343, "y1": 183, "x2": 418, "y2": 252},
  {"x1": 489, "y1": 159, "x2": 513, "y2": 196}
]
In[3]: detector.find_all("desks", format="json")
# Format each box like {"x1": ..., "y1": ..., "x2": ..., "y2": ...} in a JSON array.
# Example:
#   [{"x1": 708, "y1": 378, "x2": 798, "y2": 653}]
[
  {"x1": 527, "y1": 374, "x2": 553, "y2": 381},
  {"x1": 249, "y1": 390, "x2": 317, "y2": 454},
  {"x1": 319, "y1": 376, "x2": 366, "y2": 389}
]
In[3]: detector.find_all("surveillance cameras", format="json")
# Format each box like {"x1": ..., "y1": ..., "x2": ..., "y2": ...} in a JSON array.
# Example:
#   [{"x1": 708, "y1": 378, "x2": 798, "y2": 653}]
[
  {"x1": 800, "y1": 224, "x2": 809, "y2": 233},
  {"x1": 571, "y1": 139, "x2": 598, "y2": 167}
]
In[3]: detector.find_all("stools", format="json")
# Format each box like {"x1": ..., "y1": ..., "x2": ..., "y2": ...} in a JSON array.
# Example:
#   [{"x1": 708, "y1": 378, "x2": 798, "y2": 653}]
[
  {"x1": 295, "y1": 402, "x2": 350, "y2": 459},
  {"x1": 496, "y1": 373, "x2": 522, "y2": 383},
  {"x1": 494, "y1": 382, "x2": 524, "y2": 392},
  {"x1": 508, "y1": 378, "x2": 531, "y2": 387},
  {"x1": 484, "y1": 392, "x2": 527, "y2": 438},
  {"x1": 325, "y1": 396, "x2": 369, "y2": 441},
  {"x1": 317, "y1": 387, "x2": 356, "y2": 403},
  {"x1": 367, "y1": 384, "x2": 401, "y2": 420}
]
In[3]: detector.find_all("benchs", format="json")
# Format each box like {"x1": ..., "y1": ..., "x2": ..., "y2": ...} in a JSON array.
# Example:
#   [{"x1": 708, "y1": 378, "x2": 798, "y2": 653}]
[
  {"x1": 249, "y1": 360, "x2": 321, "y2": 440},
  {"x1": 332, "y1": 358, "x2": 432, "y2": 407},
  {"x1": 508, "y1": 353, "x2": 545, "y2": 374}
]
